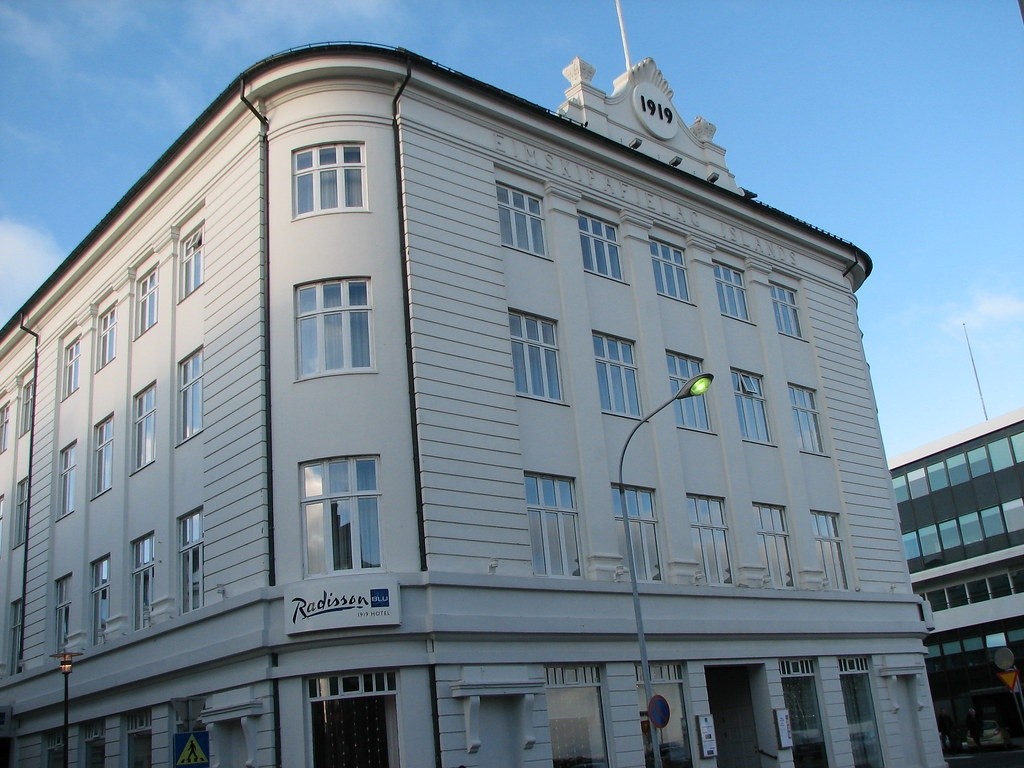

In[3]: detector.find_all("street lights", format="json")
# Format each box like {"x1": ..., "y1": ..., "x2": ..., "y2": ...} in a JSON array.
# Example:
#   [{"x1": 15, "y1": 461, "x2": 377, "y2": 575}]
[
  {"x1": 615, "y1": 373, "x2": 715, "y2": 768},
  {"x1": 50, "y1": 652, "x2": 84, "y2": 767}
]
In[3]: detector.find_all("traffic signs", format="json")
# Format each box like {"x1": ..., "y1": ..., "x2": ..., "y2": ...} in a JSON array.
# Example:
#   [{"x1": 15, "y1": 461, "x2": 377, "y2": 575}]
[{"x1": 173, "y1": 731, "x2": 210, "y2": 768}]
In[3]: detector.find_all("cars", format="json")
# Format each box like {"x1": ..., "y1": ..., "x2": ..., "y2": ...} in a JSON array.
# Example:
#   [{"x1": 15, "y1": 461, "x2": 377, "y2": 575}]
[{"x1": 966, "y1": 721, "x2": 1005, "y2": 748}]
